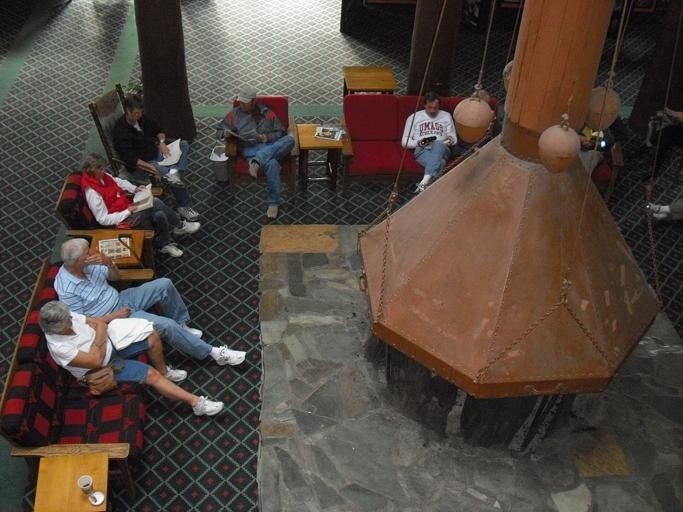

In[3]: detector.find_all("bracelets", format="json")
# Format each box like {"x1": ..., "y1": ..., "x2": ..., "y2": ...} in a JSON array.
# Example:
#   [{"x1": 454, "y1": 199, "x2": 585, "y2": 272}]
[
  {"x1": 159, "y1": 139, "x2": 165, "y2": 143},
  {"x1": 108, "y1": 261, "x2": 119, "y2": 269}
]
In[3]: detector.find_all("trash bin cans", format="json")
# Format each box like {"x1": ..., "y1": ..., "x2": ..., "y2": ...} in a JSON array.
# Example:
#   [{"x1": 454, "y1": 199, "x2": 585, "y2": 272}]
[{"x1": 209, "y1": 145, "x2": 230, "y2": 183}]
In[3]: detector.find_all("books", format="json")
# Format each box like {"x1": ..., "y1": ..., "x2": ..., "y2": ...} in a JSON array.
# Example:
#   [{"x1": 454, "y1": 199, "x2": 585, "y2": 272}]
[
  {"x1": 131, "y1": 183, "x2": 154, "y2": 214},
  {"x1": 97, "y1": 238, "x2": 130, "y2": 260},
  {"x1": 156, "y1": 138, "x2": 182, "y2": 167},
  {"x1": 315, "y1": 125, "x2": 344, "y2": 141},
  {"x1": 225, "y1": 127, "x2": 261, "y2": 144}
]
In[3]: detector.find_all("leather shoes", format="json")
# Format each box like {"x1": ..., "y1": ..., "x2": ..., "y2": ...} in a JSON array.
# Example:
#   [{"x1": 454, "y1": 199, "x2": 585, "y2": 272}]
[
  {"x1": 267, "y1": 203, "x2": 278, "y2": 219},
  {"x1": 248, "y1": 159, "x2": 260, "y2": 178}
]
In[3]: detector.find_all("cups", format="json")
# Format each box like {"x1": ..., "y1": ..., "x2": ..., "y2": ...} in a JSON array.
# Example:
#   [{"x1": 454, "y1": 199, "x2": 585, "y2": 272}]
[{"x1": 77, "y1": 475, "x2": 93, "y2": 495}]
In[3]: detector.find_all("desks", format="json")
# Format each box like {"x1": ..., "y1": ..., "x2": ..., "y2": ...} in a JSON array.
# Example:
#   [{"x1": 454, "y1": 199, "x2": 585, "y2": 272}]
[
  {"x1": 296, "y1": 123, "x2": 343, "y2": 193},
  {"x1": 339, "y1": 65, "x2": 396, "y2": 95},
  {"x1": 31, "y1": 452, "x2": 109, "y2": 511},
  {"x1": 86, "y1": 230, "x2": 146, "y2": 267}
]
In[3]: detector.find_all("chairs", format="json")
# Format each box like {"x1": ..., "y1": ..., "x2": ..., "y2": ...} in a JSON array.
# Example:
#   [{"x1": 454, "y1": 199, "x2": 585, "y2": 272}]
[
  {"x1": 53, "y1": 172, "x2": 164, "y2": 275},
  {"x1": 86, "y1": 84, "x2": 178, "y2": 182},
  {"x1": 225, "y1": 93, "x2": 289, "y2": 193},
  {"x1": 591, "y1": 137, "x2": 624, "y2": 200}
]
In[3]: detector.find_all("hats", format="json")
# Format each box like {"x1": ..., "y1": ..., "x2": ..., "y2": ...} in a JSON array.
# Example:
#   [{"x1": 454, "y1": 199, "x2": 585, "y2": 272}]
[{"x1": 237, "y1": 87, "x2": 256, "y2": 103}]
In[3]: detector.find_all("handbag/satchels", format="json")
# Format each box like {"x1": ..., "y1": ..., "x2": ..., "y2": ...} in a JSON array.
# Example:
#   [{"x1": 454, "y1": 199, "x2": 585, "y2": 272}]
[{"x1": 83, "y1": 366, "x2": 118, "y2": 395}]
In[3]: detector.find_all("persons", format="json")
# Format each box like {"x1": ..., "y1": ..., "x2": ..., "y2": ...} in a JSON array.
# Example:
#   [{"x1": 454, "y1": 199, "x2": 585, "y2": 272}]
[
  {"x1": 38, "y1": 298, "x2": 226, "y2": 418},
  {"x1": 451, "y1": 88, "x2": 498, "y2": 161},
  {"x1": 644, "y1": 106, "x2": 683, "y2": 223},
  {"x1": 79, "y1": 149, "x2": 200, "y2": 260},
  {"x1": 576, "y1": 111, "x2": 628, "y2": 200},
  {"x1": 53, "y1": 237, "x2": 248, "y2": 366},
  {"x1": 214, "y1": 84, "x2": 296, "y2": 219},
  {"x1": 400, "y1": 90, "x2": 458, "y2": 194},
  {"x1": 110, "y1": 92, "x2": 200, "y2": 222}
]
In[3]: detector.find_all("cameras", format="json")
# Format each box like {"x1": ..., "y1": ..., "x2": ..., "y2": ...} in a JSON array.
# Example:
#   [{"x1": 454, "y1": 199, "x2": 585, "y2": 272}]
[{"x1": 421, "y1": 136, "x2": 437, "y2": 145}]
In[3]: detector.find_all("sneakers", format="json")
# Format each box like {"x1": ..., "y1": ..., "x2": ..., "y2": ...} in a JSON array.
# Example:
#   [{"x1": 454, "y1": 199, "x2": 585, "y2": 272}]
[
  {"x1": 173, "y1": 222, "x2": 200, "y2": 235},
  {"x1": 192, "y1": 396, "x2": 224, "y2": 416},
  {"x1": 183, "y1": 325, "x2": 203, "y2": 338},
  {"x1": 161, "y1": 173, "x2": 184, "y2": 188},
  {"x1": 160, "y1": 243, "x2": 183, "y2": 257},
  {"x1": 216, "y1": 346, "x2": 246, "y2": 366},
  {"x1": 162, "y1": 366, "x2": 187, "y2": 382},
  {"x1": 178, "y1": 207, "x2": 200, "y2": 221}
]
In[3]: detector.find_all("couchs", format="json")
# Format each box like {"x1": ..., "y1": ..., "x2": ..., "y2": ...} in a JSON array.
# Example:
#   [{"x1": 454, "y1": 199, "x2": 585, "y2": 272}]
[
  {"x1": 0, "y1": 256, "x2": 155, "y2": 500},
  {"x1": 339, "y1": 95, "x2": 499, "y2": 178}
]
